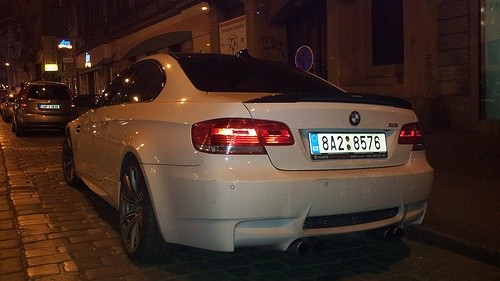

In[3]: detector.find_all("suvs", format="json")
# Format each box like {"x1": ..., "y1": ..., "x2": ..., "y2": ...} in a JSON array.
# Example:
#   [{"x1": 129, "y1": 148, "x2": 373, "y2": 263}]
[{"x1": 12, "y1": 81, "x2": 79, "y2": 137}]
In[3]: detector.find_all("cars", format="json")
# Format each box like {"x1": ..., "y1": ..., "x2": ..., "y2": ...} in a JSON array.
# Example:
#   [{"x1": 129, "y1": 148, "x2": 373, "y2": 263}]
[
  {"x1": 0, "y1": 93, "x2": 19, "y2": 122},
  {"x1": 63, "y1": 50, "x2": 435, "y2": 266}
]
